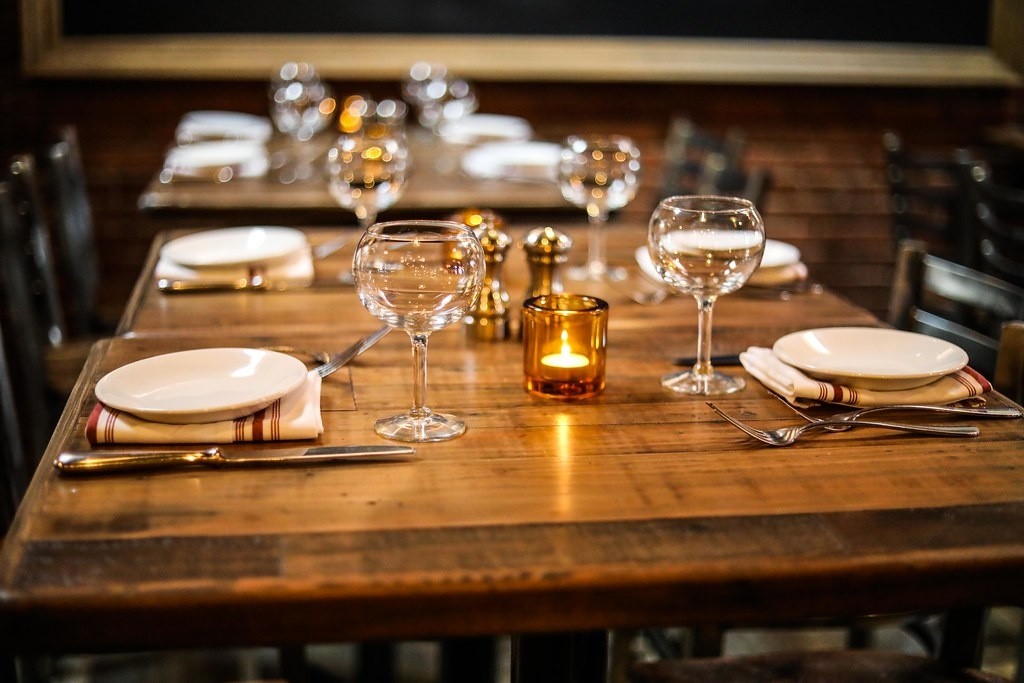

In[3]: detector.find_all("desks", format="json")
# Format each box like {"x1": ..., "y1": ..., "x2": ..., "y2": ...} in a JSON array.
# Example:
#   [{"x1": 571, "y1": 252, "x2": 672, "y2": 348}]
[
  {"x1": 136, "y1": 133, "x2": 643, "y2": 222},
  {"x1": 1, "y1": 340, "x2": 1024, "y2": 683},
  {"x1": 114, "y1": 228, "x2": 884, "y2": 338}
]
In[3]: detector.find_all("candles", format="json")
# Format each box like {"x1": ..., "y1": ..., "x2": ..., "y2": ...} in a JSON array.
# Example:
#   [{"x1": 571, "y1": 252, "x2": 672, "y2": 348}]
[{"x1": 539, "y1": 353, "x2": 589, "y2": 380}]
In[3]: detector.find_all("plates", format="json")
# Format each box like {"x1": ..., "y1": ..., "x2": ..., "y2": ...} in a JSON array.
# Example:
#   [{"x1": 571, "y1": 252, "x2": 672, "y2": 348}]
[
  {"x1": 664, "y1": 228, "x2": 800, "y2": 270},
  {"x1": 165, "y1": 110, "x2": 270, "y2": 179},
  {"x1": 157, "y1": 225, "x2": 304, "y2": 271},
  {"x1": 94, "y1": 347, "x2": 308, "y2": 426},
  {"x1": 443, "y1": 114, "x2": 532, "y2": 143},
  {"x1": 462, "y1": 141, "x2": 565, "y2": 180},
  {"x1": 772, "y1": 325, "x2": 969, "y2": 391}
]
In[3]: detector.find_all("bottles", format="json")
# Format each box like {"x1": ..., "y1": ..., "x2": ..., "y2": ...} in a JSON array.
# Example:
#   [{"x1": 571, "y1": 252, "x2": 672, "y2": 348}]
[{"x1": 464, "y1": 221, "x2": 574, "y2": 344}]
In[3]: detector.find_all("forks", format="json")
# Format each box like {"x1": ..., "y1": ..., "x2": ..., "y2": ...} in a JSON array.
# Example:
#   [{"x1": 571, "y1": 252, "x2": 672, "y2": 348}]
[
  {"x1": 765, "y1": 388, "x2": 1022, "y2": 433},
  {"x1": 252, "y1": 324, "x2": 393, "y2": 379},
  {"x1": 592, "y1": 262, "x2": 825, "y2": 307},
  {"x1": 704, "y1": 399, "x2": 980, "y2": 446}
]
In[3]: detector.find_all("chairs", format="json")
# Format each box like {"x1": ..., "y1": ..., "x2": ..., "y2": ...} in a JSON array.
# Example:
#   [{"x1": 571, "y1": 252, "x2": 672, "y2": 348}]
[
  {"x1": 0, "y1": 121, "x2": 313, "y2": 683},
  {"x1": 637, "y1": 126, "x2": 1022, "y2": 683}
]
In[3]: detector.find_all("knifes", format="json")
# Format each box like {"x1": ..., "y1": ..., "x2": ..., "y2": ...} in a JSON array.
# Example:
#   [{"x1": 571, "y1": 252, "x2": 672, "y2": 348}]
[{"x1": 51, "y1": 445, "x2": 417, "y2": 471}]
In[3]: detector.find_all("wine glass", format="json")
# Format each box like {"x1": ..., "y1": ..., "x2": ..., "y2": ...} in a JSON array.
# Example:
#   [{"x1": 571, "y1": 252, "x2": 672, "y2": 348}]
[
  {"x1": 555, "y1": 133, "x2": 642, "y2": 281},
  {"x1": 648, "y1": 194, "x2": 767, "y2": 398},
  {"x1": 352, "y1": 220, "x2": 487, "y2": 444},
  {"x1": 330, "y1": 95, "x2": 410, "y2": 285},
  {"x1": 267, "y1": 59, "x2": 330, "y2": 183}
]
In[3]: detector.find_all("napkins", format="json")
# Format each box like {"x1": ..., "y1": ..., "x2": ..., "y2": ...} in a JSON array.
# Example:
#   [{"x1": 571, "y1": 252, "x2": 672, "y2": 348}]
[
  {"x1": 739, "y1": 345, "x2": 993, "y2": 411},
  {"x1": 85, "y1": 369, "x2": 324, "y2": 446},
  {"x1": 636, "y1": 246, "x2": 808, "y2": 292},
  {"x1": 158, "y1": 246, "x2": 317, "y2": 295}
]
